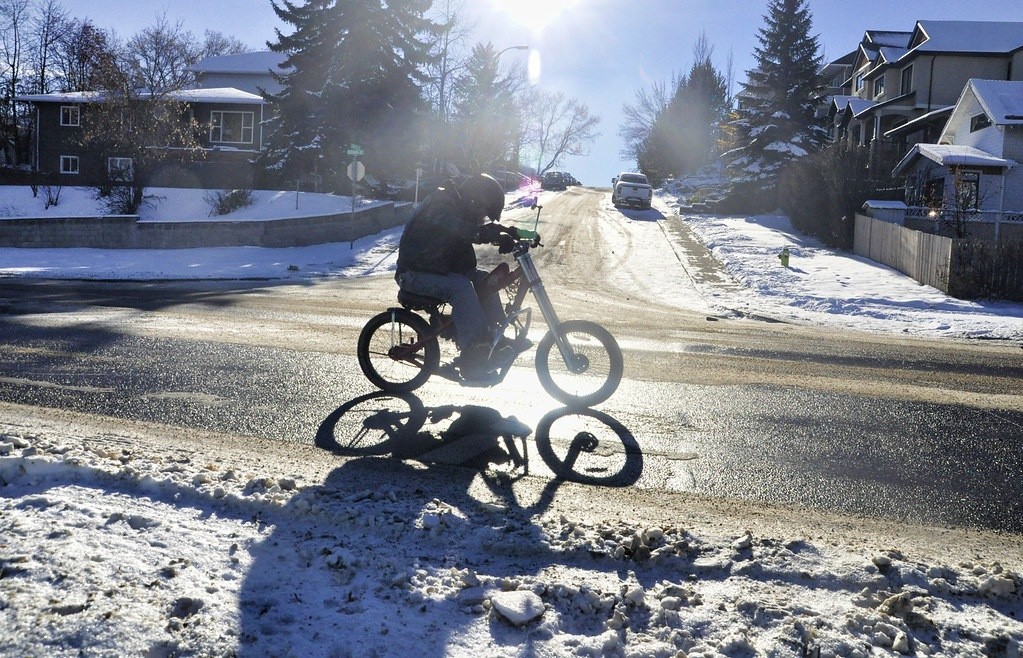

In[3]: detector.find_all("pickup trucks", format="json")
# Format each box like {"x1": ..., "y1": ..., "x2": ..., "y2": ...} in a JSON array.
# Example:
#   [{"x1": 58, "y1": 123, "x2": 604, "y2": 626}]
[
  {"x1": 541, "y1": 171, "x2": 582, "y2": 189},
  {"x1": 611, "y1": 172, "x2": 652, "y2": 208}
]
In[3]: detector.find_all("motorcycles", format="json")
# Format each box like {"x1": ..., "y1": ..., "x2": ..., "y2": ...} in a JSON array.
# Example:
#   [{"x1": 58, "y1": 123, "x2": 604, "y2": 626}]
[{"x1": 356, "y1": 196, "x2": 623, "y2": 407}]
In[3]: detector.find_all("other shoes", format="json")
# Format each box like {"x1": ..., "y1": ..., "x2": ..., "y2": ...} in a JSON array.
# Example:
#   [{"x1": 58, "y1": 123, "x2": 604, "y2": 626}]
[
  {"x1": 459, "y1": 360, "x2": 498, "y2": 382},
  {"x1": 498, "y1": 337, "x2": 533, "y2": 353}
]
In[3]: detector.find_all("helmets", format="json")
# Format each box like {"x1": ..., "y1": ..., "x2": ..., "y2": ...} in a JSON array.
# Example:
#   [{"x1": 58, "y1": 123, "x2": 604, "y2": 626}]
[{"x1": 459, "y1": 172, "x2": 505, "y2": 222}]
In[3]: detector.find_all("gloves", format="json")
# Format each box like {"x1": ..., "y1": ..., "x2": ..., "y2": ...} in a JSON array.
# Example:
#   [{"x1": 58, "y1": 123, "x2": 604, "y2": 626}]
[
  {"x1": 514, "y1": 227, "x2": 541, "y2": 248},
  {"x1": 488, "y1": 230, "x2": 515, "y2": 254}
]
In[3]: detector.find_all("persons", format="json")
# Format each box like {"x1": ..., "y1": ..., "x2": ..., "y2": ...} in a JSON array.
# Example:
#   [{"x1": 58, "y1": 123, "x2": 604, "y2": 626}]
[{"x1": 395, "y1": 173, "x2": 541, "y2": 379}]
